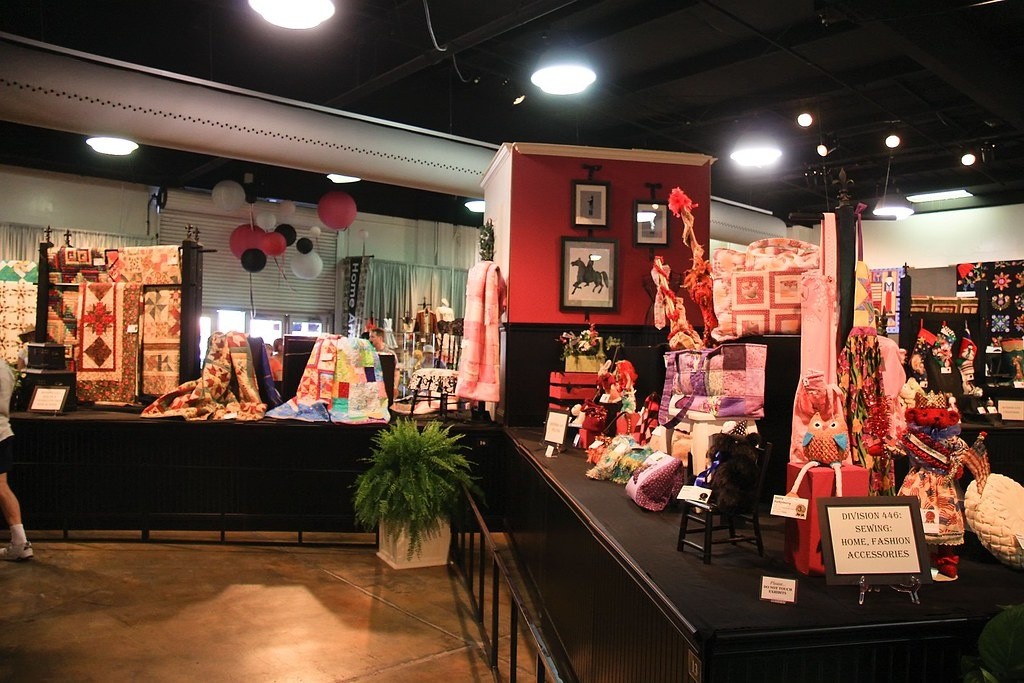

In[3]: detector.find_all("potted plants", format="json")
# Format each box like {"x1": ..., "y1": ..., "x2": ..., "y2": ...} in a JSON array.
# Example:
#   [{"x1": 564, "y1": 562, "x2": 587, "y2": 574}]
[{"x1": 349, "y1": 416, "x2": 484, "y2": 570}]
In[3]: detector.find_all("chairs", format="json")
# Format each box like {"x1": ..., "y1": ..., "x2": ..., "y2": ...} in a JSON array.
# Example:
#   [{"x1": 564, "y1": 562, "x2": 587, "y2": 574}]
[{"x1": 677, "y1": 444, "x2": 766, "y2": 563}]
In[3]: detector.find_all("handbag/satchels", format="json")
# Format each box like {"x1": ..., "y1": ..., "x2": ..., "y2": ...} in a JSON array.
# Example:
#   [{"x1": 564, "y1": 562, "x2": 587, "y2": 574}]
[
  {"x1": 709, "y1": 238, "x2": 820, "y2": 341},
  {"x1": 579, "y1": 398, "x2": 685, "y2": 511},
  {"x1": 658, "y1": 343, "x2": 768, "y2": 426}
]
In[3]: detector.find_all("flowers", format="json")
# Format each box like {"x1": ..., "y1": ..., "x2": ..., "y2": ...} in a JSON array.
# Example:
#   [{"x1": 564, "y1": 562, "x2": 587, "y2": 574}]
[{"x1": 556, "y1": 322, "x2": 624, "y2": 362}]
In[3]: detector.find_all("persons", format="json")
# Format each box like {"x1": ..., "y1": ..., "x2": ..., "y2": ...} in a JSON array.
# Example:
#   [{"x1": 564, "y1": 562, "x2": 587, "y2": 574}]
[
  {"x1": 369, "y1": 327, "x2": 399, "y2": 398},
  {"x1": 615, "y1": 360, "x2": 638, "y2": 414},
  {"x1": 0, "y1": 359, "x2": 33, "y2": 559},
  {"x1": 273, "y1": 337, "x2": 283, "y2": 365},
  {"x1": 407, "y1": 349, "x2": 422, "y2": 380},
  {"x1": 415, "y1": 345, "x2": 446, "y2": 370},
  {"x1": 265, "y1": 344, "x2": 282, "y2": 380},
  {"x1": 436, "y1": 297, "x2": 458, "y2": 363}
]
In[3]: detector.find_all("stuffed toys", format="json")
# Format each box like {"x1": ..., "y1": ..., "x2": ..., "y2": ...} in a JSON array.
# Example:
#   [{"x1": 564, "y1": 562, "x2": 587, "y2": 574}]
[
  {"x1": 897, "y1": 390, "x2": 969, "y2": 579},
  {"x1": 786, "y1": 412, "x2": 849, "y2": 497},
  {"x1": 597, "y1": 360, "x2": 622, "y2": 403}
]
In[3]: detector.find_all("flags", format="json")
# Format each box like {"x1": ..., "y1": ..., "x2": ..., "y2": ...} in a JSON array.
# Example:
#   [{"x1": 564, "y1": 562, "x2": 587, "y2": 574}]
[{"x1": 962, "y1": 439, "x2": 990, "y2": 499}]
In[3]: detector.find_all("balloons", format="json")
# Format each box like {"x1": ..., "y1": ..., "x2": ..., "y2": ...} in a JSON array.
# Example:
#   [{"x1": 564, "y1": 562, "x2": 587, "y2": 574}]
[
  {"x1": 357, "y1": 229, "x2": 368, "y2": 239},
  {"x1": 229, "y1": 224, "x2": 266, "y2": 260},
  {"x1": 262, "y1": 232, "x2": 286, "y2": 256},
  {"x1": 256, "y1": 211, "x2": 276, "y2": 229},
  {"x1": 274, "y1": 224, "x2": 323, "y2": 278},
  {"x1": 279, "y1": 200, "x2": 295, "y2": 217},
  {"x1": 241, "y1": 248, "x2": 266, "y2": 272},
  {"x1": 212, "y1": 179, "x2": 244, "y2": 212},
  {"x1": 317, "y1": 190, "x2": 356, "y2": 229}
]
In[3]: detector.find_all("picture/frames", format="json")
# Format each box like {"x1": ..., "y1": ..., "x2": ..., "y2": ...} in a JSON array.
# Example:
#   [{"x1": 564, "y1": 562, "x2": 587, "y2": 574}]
[
  {"x1": 632, "y1": 200, "x2": 669, "y2": 248},
  {"x1": 818, "y1": 493, "x2": 932, "y2": 587},
  {"x1": 570, "y1": 179, "x2": 611, "y2": 230},
  {"x1": 559, "y1": 235, "x2": 618, "y2": 313},
  {"x1": 28, "y1": 386, "x2": 70, "y2": 415}
]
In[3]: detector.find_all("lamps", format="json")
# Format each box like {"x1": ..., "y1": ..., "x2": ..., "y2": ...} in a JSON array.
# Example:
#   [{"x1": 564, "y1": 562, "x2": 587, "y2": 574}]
[
  {"x1": 465, "y1": 199, "x2": 485, "y2": 212},
  {"x1": 147, "y1": 185, "x2": 168, "y2": 235},
  {"x1": 531, "y1": 23, "x2": 597, "y2": 94},
  {"x1": 327, "y1": 174, "x2": 361, "y2": 183},
  {"x1": 731, "y1": 110, "x2": 987, "y2": 215},
  {"x1": 86, "y1": 136, "x2": 139, "y2": 156},
  {"x1": 248, "y1": 0, "x2": 337, "y2": 30}
]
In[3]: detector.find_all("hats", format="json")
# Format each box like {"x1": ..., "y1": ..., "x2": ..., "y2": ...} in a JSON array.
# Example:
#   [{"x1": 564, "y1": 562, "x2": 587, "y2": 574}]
[{"x1": 423, "y1": 345, "x2": 432, "y2": 353}]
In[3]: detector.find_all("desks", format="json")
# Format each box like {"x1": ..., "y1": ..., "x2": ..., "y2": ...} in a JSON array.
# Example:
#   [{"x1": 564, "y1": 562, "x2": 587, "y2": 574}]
[{"x1": 4, "y1": 405, "x2": 1023, "y2": 683}]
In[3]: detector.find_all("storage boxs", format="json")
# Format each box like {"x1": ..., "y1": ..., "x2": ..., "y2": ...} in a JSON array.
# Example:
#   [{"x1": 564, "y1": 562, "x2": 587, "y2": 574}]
[
  {"x1": 24, "y1": 343, "x2": 77, "y2": 410},
  {"x1": 549, "y1": 370, "x2": 599, "y2": 411}
]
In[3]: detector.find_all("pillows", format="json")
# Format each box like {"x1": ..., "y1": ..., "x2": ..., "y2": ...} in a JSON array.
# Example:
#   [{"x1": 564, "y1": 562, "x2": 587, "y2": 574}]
[{"x1": 711, "y1": 238, "x2": 820, "y2": 343}]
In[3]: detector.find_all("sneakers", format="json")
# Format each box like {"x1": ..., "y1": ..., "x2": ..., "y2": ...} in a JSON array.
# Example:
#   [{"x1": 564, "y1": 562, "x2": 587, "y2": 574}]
[{"x1": 0, "y1": 541, "x2": 33, "y2": 560}]
